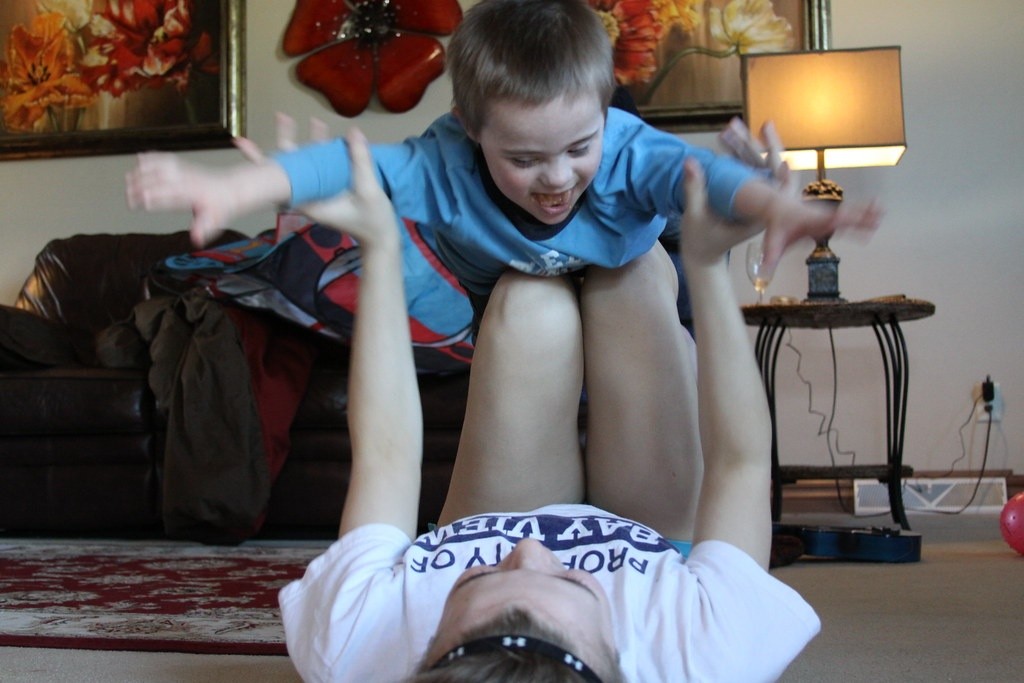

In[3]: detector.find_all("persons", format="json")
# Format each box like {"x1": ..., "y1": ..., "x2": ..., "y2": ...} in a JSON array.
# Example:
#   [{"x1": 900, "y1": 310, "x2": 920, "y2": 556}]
[
  {"x1": 233, "y1": 111, "x2": 821, "y2": 683},
  {"x1": 126, "y1": 0, "x2": 888, "y2": 347}
]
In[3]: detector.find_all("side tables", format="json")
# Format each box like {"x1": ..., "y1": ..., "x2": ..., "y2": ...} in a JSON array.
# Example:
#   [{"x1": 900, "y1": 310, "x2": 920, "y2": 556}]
[{"x1": 738, "y1": 297, "x2": 936, "y2": 531}]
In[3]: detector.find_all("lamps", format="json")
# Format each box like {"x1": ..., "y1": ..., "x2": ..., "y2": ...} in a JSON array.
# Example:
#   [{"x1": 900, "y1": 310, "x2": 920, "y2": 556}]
[{"x1": 738, "y1": 45, "x2": 908, "y2": 306}]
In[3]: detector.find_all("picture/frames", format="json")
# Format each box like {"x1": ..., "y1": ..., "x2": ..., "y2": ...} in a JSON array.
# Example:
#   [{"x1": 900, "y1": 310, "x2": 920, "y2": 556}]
[
  {"x1": 0, "y1": 0, "x2": 248, "y2": 162},
  {"x1": 586, "y1": 0, "x2": 832, "y2": 132}
]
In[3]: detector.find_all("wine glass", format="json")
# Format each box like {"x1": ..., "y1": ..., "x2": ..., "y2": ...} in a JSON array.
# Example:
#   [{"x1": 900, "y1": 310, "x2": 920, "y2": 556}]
[{"x1": 745, "y1": 243, "x2": 775, "y2": 306}]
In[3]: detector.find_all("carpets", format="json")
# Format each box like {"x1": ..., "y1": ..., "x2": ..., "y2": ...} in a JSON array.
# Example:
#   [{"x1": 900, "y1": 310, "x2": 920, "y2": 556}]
[{"x1": 0, "y1": 538, "x2": 329, "y2": 656}]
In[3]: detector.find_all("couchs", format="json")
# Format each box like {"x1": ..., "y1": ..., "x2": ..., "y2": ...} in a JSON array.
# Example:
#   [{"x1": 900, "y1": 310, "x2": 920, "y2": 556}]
[{"x1": 0, "y1": 230, "x2": 587, "y2": 538}]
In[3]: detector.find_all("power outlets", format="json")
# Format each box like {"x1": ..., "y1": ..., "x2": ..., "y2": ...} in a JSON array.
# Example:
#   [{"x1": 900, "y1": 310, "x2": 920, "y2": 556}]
[{"x1": 974, "y1": 380, "x2": 1003, "y2": 421}]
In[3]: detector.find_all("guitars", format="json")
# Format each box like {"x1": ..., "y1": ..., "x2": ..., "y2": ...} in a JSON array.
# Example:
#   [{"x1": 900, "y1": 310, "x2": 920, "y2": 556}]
[{"x1": 772, "y1": 524, "x2": 922, "y2": 563}]
